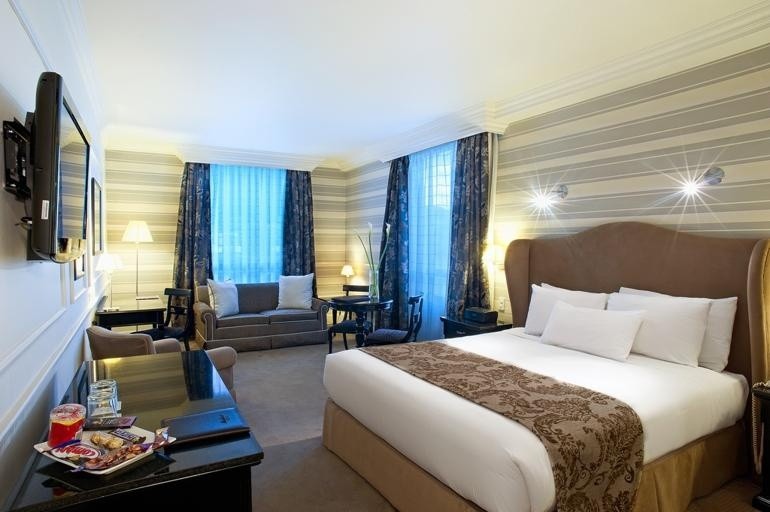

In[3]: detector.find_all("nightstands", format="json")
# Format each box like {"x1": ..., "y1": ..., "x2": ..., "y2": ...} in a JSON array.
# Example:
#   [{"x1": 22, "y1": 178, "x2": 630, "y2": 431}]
[
  {"x1": 752, "y1": 386, "x2": 770, "y2": 510},
  {"x1": 440, "y1": 316, "x2": 514, "y2": 338}
]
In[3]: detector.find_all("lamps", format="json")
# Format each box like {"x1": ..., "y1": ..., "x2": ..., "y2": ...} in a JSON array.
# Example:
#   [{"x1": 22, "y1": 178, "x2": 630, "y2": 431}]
[
  {"x1": 492, "y1": 247, "x2": 506, "y2": 273},
  {"x1": 683, "y1": 168, "x2": 726, "y2": 195},
  {"x1": 341, "y1": 266, "x2": 355, "y2": 279},
  {"x1": 122, "y1": 221, "x2": 154, "y2": 295},
  {"x1": 537, "y1": 185, "x2": 568, "y2": 208}
]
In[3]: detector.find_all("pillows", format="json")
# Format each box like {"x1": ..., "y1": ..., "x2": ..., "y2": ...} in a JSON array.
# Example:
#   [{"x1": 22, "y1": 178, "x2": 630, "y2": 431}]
[
  {"x1": 607, "y1": 294, "x2": 711, "y2": 368},
  {"x1": 541, "y1": 302, "x2": 647, "y2": 362},
  {"x1": 277, "y1": 273, "x2": 315, "y2": 311},
  {"x1": 619, "y1": 287, "x2": 739, "y2": 374},
  {"x1": 524, "y1": 282, "x2": 609, "y2": 337},
  {"x1": 207, "y1": 279, "x2": 240, "y2": 319}
]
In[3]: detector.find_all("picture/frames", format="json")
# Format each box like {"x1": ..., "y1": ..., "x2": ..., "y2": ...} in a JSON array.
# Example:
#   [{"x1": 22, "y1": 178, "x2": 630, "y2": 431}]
[
  {"x1": 92, "y1": 179, "x2": 106, "y2": 257},
  {"x1": 75, "y1": 255, "x2": 88, "y2": 281}
]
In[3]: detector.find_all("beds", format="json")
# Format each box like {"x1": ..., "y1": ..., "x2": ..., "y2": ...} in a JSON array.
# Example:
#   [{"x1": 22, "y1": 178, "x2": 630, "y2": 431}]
[{"x1": 323, "y1": 221, "x2": 770, "y2": 512}]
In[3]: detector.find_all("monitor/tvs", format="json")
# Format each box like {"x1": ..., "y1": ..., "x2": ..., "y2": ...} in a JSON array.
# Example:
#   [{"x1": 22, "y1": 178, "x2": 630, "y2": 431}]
[{"x1": 24, "y1": 72, "x2": 90, "y2": 262}]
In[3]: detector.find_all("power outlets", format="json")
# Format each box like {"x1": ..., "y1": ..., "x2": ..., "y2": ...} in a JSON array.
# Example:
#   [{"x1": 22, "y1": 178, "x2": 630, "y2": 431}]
[{"x1": 499, "y1": 300, "x2": 506, "y2": 312}]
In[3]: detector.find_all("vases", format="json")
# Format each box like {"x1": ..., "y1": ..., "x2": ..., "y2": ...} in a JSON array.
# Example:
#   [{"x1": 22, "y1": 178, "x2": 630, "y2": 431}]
[{"x1": 354, "y1": 222, "x2": 392, "y2": 299}]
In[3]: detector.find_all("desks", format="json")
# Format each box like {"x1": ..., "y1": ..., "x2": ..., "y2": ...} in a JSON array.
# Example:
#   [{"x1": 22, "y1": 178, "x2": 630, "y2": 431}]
[
  {"x1": 96, "y1": 294, "x2": 167, "y2": 331},
  {"x1": 328, "y1": 297, "x2": 395, "y2": 348},
  {"x1": 0, "y1": 350, "x2": 265, "y2": 511}
]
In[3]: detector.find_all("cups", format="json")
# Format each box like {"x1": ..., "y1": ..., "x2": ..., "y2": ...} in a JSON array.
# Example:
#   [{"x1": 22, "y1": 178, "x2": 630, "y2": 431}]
[{"x1": 87, "y1": 380, "x2": 118, "y2": 419}]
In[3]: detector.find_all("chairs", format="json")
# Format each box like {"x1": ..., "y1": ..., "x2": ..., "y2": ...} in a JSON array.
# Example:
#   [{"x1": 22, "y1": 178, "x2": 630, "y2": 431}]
[
  {"x1": 329, "y1": 286, "x2": 375, "y2": 355},
  {"x1": 364, "y1": 292, "x2": 424, "y2": 346},
  {"x1": 131, "y1": 289, "x2": 194, "y2": 351}
]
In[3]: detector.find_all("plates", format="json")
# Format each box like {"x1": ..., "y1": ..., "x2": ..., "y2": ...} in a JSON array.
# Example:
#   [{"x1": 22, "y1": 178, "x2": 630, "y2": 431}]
[{"x1": 33, "y1": 425, "x2": 177, "y2": 475}]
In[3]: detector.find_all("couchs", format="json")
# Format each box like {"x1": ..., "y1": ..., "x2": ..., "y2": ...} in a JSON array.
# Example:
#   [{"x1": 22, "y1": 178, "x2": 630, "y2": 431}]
[
  {"x1": 194, "y1": 283, "x2": 330, "y2": 353},
  {"x1": 86, "y1": 326, "x2": 238, "y2": 403}
]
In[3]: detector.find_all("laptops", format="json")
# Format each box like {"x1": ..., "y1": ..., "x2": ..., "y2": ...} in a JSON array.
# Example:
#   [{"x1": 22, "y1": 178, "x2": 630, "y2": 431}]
[{"x1": 331, "y1": 296, "x2": 370, "y2": 303}]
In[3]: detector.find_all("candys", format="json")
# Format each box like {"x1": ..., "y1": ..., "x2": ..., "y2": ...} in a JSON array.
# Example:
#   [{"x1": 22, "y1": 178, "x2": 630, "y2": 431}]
[
  {"x1": 70, "y1": 457, "x2": 111, "y2": 473},
  {"x1": 91, "y1": 431, "x2": 122, "y2": 449},
  {"x1": 109, "y1": 454, "x2": 128, "y2": 465},
  {"x1": 111, "y1": 443, "x2": 129, "y2": 461},
  {"x1": 125, "y1": 453, "x2": 138, "y2": 459},
  {"x1": 130, "y1": 443, "x2": 154, "y2": 454},
  {"x1": 103, "y1": 450, "x2": 117, "y2": 463}
]
